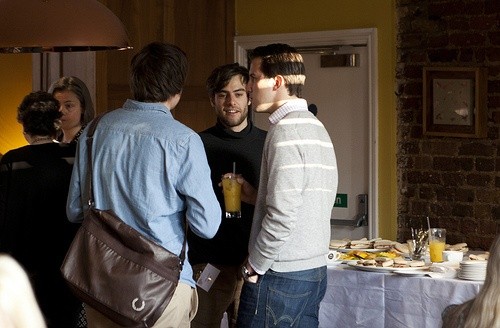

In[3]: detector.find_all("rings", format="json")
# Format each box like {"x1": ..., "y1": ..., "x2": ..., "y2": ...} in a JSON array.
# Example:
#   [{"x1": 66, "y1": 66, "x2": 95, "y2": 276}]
[{"x1": 199, "y1": 270, "x2": 202, "y2": 274}]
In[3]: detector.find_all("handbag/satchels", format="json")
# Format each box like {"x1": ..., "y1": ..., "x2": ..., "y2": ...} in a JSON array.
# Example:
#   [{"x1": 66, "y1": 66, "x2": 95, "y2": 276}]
[{"x1": 59, "y1": 206, "x2": 184, "y2": 328}]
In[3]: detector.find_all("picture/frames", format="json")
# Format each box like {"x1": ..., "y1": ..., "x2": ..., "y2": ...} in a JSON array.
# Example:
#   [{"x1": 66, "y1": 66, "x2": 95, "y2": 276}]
[{"x1": 423, "y1": 67, "x2": 488, "y2": 139}]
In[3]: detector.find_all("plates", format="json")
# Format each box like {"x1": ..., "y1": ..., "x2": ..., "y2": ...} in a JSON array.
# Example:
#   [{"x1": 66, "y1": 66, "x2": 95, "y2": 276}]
[
  {"x1": 393, "y1": 271, "x2": 434, "y2": 277},
  {"x1": 456, "y1": 260, "x2": 488, "y2": 281},
  {"x1": 329, "y1": 245, "x2": 347, "y2": 249},
  {"x1": 326, "y1": 260, "x2": 430, "y2": 273},
  {"x1": 338, "y1": 248, "x2": 387, "y2": 253}
]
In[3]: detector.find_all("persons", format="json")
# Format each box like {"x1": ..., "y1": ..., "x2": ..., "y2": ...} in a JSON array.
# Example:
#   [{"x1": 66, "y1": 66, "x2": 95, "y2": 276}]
[
  {"x1": 218, "y1": 43, "x2": 339, "y2": 328},
  {"x1": 186, "y1": 62, "x2": 268, "y2": 328},
  {"x1": 66, "y1": 41, "x2": 223, "y2": 328},
  {"x1": 0, "y1": 76, "x2": 94, "y2": 328},
  {"x1": 439, "y1": 234, "x2": 500, "y2": 328}
]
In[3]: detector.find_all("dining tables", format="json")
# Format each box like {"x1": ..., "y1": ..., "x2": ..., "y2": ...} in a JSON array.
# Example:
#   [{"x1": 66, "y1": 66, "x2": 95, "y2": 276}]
[{"x1": 319, "y1": 240, "x2": 489, "y2": 328}]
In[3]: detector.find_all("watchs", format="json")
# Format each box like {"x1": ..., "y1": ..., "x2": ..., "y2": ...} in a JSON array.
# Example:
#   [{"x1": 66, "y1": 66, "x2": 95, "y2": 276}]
[{"x1": 240, "y1": 260, "x2": 258, "y2": 278}]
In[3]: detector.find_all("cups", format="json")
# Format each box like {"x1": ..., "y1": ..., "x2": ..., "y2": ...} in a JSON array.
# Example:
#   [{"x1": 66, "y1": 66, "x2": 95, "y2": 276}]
[
  {"x1": 428, "y1": 229, "x2": 446, "y2": 263},
  {"x1": 222, "y1": 174, "x2": 242, "y2": 218},
  {"x1": 442, "y1": 251, "x2": 463, "y2": 263},
  {"x1": 407, "y1": 240, "x2": 428, "y2": 262}
]
